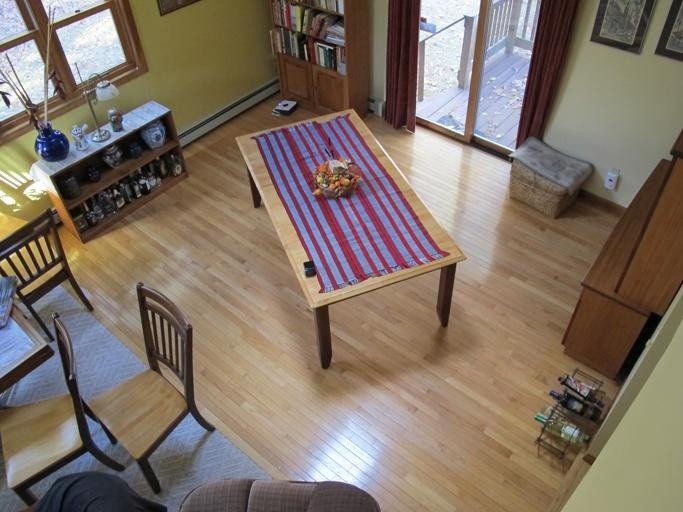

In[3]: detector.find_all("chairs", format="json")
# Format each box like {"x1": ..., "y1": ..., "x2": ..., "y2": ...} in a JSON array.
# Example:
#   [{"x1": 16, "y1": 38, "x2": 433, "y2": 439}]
[
  {"x1": 0, "y1": 205, "x2": 95, "y2": 342},
  {"x1": 83, "y1": 281, "x2": 216, "y2": 495},
  {"x1": 0, "y1": 311, "x2": 127, "y2": 507}
]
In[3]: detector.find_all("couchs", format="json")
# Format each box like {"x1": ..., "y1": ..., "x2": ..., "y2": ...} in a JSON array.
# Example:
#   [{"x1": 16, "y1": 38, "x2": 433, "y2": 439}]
[{"x1": 177, "y1": 476, "x2": 381, "y2": 512}]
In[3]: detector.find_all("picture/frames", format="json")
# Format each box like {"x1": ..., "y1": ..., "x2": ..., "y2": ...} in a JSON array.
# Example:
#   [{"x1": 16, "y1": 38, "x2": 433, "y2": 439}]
[
  {"x1": 157, "y1": 0, "x2": 201, "y2": 16},
  {"x1": 589, "y1": 0, "x2": 656, "y2": 54},
  {"x1": 654, "y1": 0, "x2": 683, "y2": 60}
]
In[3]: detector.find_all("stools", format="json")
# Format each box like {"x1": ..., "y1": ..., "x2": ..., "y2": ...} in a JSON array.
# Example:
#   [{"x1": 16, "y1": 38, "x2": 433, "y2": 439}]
[{"x1": 508, "y1": 136, "x2": 594, "y2": 219}]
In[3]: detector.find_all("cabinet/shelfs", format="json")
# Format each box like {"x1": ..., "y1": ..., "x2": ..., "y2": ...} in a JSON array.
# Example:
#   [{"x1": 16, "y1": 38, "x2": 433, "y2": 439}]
[
  {"x1": 268, "y1": 0, "x2": 370, "y2": 119},
  {"x1": 560, "y1": 126, "x2": 683, "y2": 381},
  {"x1": 28, "y1": 99, "x2": 190, "y2": 245}
]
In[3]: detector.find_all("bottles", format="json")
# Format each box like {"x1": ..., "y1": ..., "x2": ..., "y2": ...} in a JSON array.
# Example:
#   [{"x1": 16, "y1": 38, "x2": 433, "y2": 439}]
[
  {"x1": 534, "y1": 411, "x2": 590, "y2": 449},
  {"x1": 559, "y1": 373, "x2": 606, "y2": 409},
  {"x1": 91, "y1": 196, "x2": 104, "y2": 220},
  {"x1": 120, "y1": 183, "x2": 131, "y2": 204},
  {"x1": 168, "y1": 154, "x2": 182, "y2": 176},
  {"x1": 147, "y1": 172, "x2": 156, "y2": 187},
  {"x1": 137, "y1": 167, "x2": 151, "y2": 195},
  {"x1": 129, "y1": 173, "x2": 142, "y2": 199},
  {"x1": 549, "y1": 389, "x2": 603, "y2": 424},
  {"x1": 156, "y1": 156, "x2": 168, "y2": 178},
  {"x1": 112, "y1": 185, "x2": 126, "y2": 209},
  {"x1": 82, "y1": 201, "x2": 98, "y2": 226}
]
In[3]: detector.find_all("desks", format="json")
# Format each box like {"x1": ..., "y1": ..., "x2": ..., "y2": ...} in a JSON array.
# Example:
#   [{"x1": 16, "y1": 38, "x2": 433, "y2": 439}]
[{"x1": 233, "y1": 108, "x2": 468, "y2": 369}]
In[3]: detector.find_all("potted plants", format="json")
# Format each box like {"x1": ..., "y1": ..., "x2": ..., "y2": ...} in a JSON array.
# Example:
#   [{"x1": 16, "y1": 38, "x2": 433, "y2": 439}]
[{"x1": 1, "y1": 6, "x2": 70, "y2": 163}]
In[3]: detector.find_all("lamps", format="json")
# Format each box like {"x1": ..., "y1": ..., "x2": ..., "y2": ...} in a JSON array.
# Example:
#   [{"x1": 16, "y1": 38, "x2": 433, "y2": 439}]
[{"x1": 74, "y1": 61, "x2": 120, "y2": 143}]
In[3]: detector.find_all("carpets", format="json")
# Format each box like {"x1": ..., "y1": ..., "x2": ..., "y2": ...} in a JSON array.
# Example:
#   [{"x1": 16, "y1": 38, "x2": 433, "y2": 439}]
[{"x1": 0, "y1": 284, "x2": 274, "y2": 512}]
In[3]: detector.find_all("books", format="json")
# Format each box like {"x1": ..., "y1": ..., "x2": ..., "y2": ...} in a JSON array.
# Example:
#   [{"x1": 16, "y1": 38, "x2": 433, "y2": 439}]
[
  {"x1": 269, "y1": 27, "x2": 346, "y2": 76},
  {"x1": 272, "y1": 0, "x2": 345, "y2": 47},
  {"x1": 296, "y1": 0, "x2": 345, "y2": 13}
]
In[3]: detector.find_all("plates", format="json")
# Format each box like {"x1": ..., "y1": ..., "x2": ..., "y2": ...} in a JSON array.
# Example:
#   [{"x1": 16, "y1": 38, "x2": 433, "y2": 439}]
[{"x1": 317, "y1": 162, "x2": 361, "y2": 199}]
